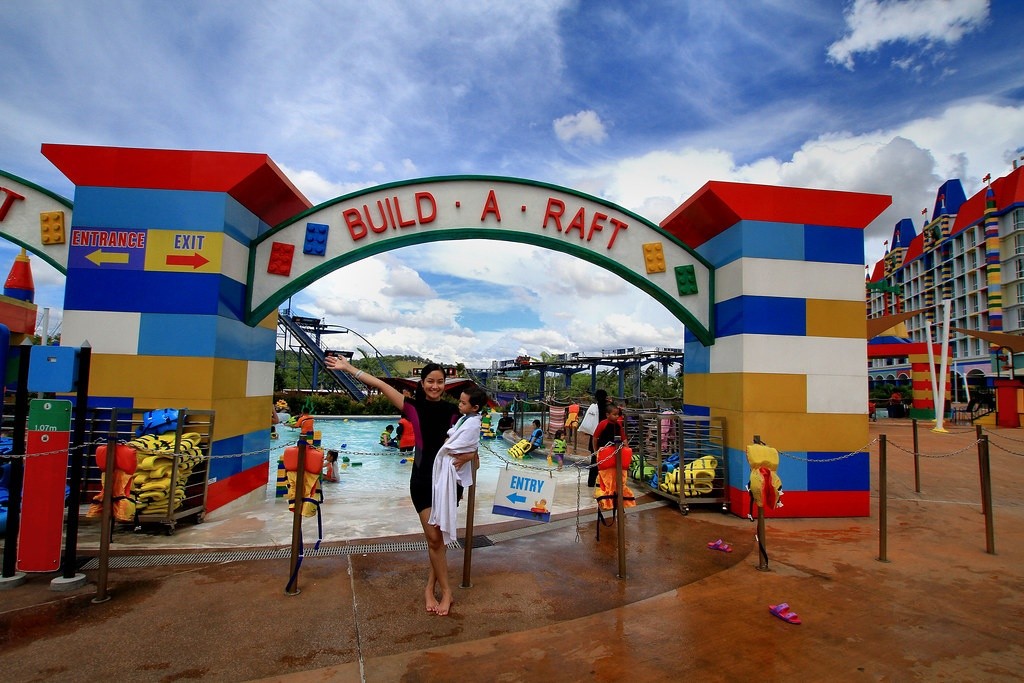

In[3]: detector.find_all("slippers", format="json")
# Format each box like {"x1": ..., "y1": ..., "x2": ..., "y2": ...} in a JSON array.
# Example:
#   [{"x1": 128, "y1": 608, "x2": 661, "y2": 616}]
[
  {"x1": 708, "y1": 539, "x2": 732, "y2": 553},
  {"x1": 769, "y1": 603, "x2": 801, "y2": 624}
]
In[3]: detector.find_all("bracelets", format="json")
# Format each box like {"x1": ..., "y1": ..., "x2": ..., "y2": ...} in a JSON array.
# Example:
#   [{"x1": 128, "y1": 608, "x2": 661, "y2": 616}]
[{"x1": 355, "y1": 370, "x2": 363, "y2": 379}]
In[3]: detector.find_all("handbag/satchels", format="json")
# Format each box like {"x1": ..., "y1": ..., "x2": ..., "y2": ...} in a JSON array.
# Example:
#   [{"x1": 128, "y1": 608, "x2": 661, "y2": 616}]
[{"x1": 577, "y1": 404, "x2": 599, "y2": 436}]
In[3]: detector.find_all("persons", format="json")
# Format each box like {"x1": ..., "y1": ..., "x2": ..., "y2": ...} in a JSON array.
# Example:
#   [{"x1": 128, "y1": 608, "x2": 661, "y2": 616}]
[
  {"x1": 445, "y1": 389, "x2": 487, "y2": 473},
  {"x1": 271, "y1": 405, "x2": 279, "y2": 433},
  {"x1": 587, "y1": 390, "x2": 628, "y2": 487},
  {"x1": 890, "y1": 388, "x2": 902, "y2": 403},
  {"x1": 549, "y1": 430, "x2": 569, "y2": 471},
  {"x1": 497, "y1": 411, "x2": 514, "y2": 434},
  {"x1": 869, "y1": 400, "x2": 876, "y2": 422},
  {"x1": 481, "y1": 410, "x2": 488, "y2": 417},
  {"x1": 396, "y1": 412, "x2": 416, "y2": 455},
  {"x1": 294, "y1": 407, "x2": 314, "y2": 436},
  {"x1": 380, "y1": 425, "x2": 397, "y2": 448},
  {"x1": 322, "y1": 450, "x2": 340, "y2": 482},
  {"x1": 323, "y1": 354, "x2": 476, "y2": 617},
  {"x1": 525, "y1": 420, "x2": 543, "y2": 456}
]
